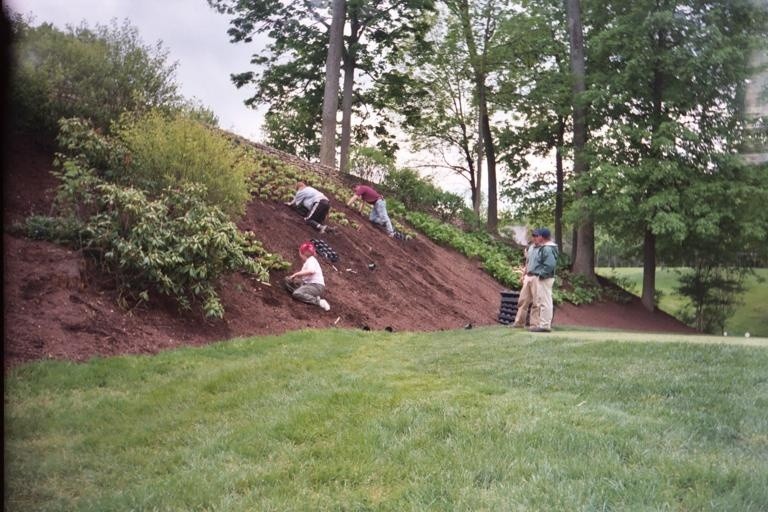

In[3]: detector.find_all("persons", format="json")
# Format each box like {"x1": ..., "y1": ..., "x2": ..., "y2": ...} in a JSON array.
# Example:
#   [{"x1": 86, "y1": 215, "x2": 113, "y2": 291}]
[
  {"x1": 285, "y1": 241, "x2": 330, "y2": 313},
  {"x1": 510, "y1": 230, "x2": 543, "y2": 329},
  {"x1": 346, "y1": 183, "x2": 395, "y2": 238},
  {"x1": 532, "y1": 227, "x2": 559, "y2": 331},
  {"x1": 524, "y1": 244, "x2": 529, "y2": 257},
  {"x1": 284, "y1": 180, "x2": 338, "y2": 237}
]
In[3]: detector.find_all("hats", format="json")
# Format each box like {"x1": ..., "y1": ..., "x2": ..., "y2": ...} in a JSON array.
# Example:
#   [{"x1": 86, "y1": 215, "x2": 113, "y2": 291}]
[
  {"x1": 301, "y1": 241, "x2": 316, "y2": 255},
  {"x1": 532, "y1": 228, "x2": 551, "y2": 238}
]
